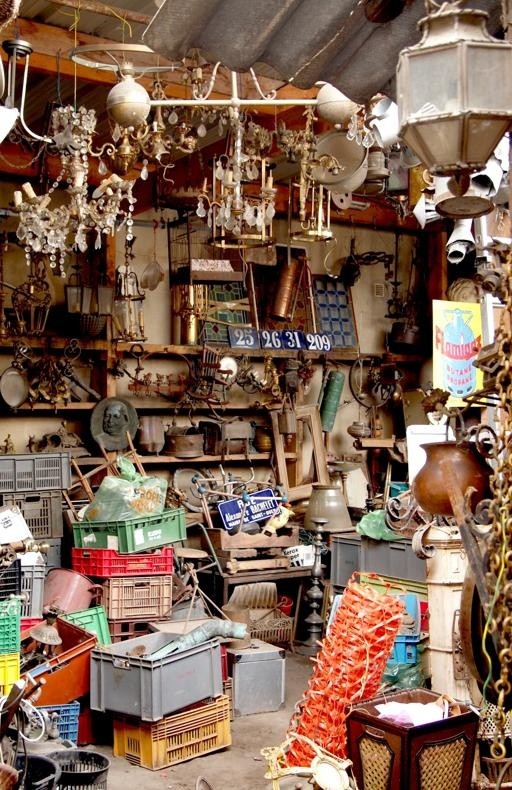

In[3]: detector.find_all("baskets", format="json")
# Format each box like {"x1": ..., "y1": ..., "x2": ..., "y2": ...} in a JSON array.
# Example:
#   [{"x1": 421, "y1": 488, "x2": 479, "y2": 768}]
[{"x1": 0, "y1": 453, "x2": 234, "y2": 770}]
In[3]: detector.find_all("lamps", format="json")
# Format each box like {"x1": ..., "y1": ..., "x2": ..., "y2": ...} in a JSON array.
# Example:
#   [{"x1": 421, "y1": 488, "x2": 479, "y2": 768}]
[
  {"x1": 316, "y1": 81, "x2": 358, "y2": 132},
  {"x1": 161, "y1": 62, "x2": 238, "y2": 134},
  {"x1": 67, "y1": 41, "x2": 188, "y2": 130},
  {"x1": 284, "y1": 105, "x2": 334, "y2": 246},
  {"x1": 397, "y1": 1, "x2": 512, "y2": 215},
  {"x1": 101, "y1": 73, "x2": 198, "y2": 185},
  {"x1": 194, "y1": 117, "x2": 280, "y2": 253},
  {"x1": 13, "y1": 102, "x2": 137, "y2": 282}
]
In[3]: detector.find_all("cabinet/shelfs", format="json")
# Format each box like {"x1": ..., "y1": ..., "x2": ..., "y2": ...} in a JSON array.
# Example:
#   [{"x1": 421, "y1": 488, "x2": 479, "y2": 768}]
[{"x1": 1, "y1": 332, "x2": 360, "y2": 493}]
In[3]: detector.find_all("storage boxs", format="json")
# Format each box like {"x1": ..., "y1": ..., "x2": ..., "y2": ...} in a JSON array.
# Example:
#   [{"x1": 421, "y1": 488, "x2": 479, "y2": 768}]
[
  {"x1": 20, "y1": 618, "x2": 97, "y2": 707},
  {"x1": 228, "y1": 639, "x2": 286, "y2": 717},
  {"x1": 330, "y1": 534, "x2": 426, "y2": 665},
  {"x1": 89, "y1": 630, "x2": 223, "y2": 723},
  {"x1": 70, "y1": 504, "x2": 186, "y2": 553}
]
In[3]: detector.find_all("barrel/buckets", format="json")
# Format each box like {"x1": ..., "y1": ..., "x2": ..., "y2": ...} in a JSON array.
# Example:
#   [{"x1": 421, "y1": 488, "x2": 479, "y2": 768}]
[{"x1": 304, "y1": 485, "x2": 353, "y2": 532}]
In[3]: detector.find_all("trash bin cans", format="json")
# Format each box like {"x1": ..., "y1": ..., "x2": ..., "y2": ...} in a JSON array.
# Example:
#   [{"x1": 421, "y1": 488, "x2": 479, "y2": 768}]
[{"x1": 346, "y1": 687, "x2": 479, "y2": 790}]
